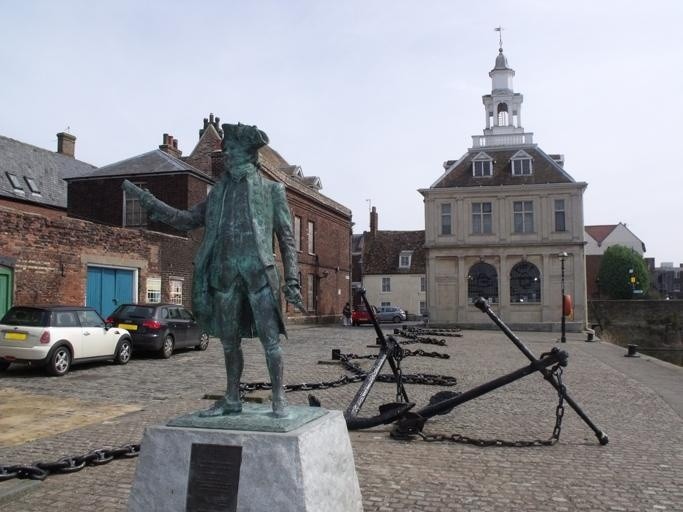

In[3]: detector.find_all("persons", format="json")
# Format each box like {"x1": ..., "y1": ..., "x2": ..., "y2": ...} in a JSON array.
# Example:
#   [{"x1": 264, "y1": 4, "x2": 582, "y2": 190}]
[
  {"x1": 122, "y1": 122, "x2": 306, "y2": 419},
  {"x1": 343, "y1": 302, "x2": 352, "y2": 327}
]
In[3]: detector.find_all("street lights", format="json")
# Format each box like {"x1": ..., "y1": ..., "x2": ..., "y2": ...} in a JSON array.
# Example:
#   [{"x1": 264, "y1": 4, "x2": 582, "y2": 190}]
[{"x1": 554, "y1": 249, "x2": 568, "y2": 342}]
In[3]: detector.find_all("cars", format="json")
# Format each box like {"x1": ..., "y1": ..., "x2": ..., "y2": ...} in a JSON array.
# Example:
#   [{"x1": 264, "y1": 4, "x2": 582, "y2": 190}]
[
  {"x1": 376, "y1": 304, "x2": 407, "y2": 323},
  {"x1": 0, "y1": 303, "x2": 133, "y2": 378},
  {"x1": 102, "y1": 301, "x2": 211, "y2": 359},
  {"x1": 351, "y1": 304, "x2": 378, "y2": 326}
]
